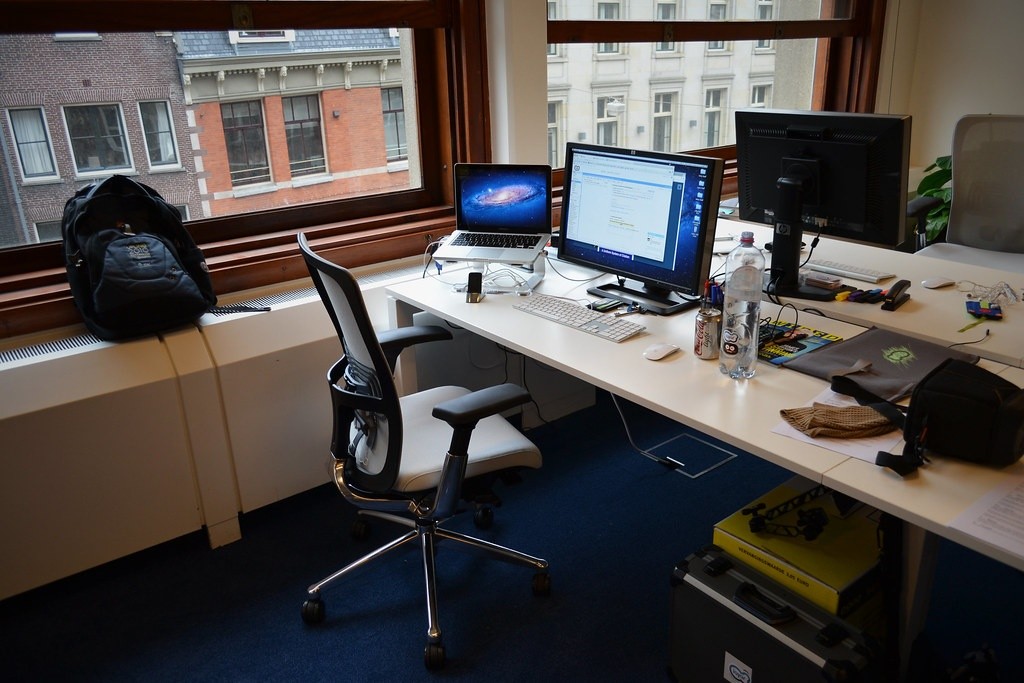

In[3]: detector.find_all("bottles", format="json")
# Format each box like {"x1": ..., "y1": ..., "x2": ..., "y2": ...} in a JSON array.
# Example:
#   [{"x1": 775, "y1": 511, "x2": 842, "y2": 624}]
[{"x1": 719, "y1": 231, "x2": 766, "y2": 381}]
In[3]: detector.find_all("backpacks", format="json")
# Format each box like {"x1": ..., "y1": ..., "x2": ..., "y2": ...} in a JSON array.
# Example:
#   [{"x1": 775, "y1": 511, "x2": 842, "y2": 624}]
[{"x1": 60, "y1": 175, "x2": 270, "y2": 346}]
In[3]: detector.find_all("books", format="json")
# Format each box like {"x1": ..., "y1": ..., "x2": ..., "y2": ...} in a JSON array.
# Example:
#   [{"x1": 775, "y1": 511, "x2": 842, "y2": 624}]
[{"x1": 756, "y1": 320, "x2": 843, "y2": 370}]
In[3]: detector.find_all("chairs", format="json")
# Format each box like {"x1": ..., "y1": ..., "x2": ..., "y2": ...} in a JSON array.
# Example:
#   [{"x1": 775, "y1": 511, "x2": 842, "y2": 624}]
[{"x1": 292, "y1": 232, "x2": 553, "y2": 670}]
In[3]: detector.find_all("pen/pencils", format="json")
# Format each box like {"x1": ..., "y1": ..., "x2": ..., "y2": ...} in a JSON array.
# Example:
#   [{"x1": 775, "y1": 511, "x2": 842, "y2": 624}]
[
  {"x1": 703, "y1": 277, "x2": 724, "y2": 305},
  {"x1": 765, "y1": 333, "x2": 808, "y2": 346}
]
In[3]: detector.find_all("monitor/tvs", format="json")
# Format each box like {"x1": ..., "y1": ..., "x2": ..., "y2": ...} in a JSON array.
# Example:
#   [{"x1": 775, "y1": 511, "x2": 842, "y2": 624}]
[
  {"x1": 557, "y1": 142, "x2": 725, "y2": 315},
  {"x1": 735, "y1": 109, "x2": 912, "y2": 300}
]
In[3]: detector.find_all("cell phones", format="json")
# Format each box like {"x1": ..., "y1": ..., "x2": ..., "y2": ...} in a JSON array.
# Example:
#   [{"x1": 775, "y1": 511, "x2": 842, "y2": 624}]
[{"x1": 468, "y1": 273, "x2": 482, "y2": 294}]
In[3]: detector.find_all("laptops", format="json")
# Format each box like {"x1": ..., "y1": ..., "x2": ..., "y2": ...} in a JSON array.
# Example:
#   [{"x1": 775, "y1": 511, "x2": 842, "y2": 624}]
[{"x1": 432, "y1": 163, "x2": 552, "y2": 264}]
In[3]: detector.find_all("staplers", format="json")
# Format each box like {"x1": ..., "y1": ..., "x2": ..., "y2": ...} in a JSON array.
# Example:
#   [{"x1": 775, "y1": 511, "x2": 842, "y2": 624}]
[{"x1": 880, "y1": 279, "x2": 911, "y2": 312}]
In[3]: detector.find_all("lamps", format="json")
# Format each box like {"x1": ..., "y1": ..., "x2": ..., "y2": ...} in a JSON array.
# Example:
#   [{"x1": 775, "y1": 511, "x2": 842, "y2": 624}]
[
  {"x1": 636, "y1": 126, "x2": 644, "y2": 133},
  {"x1": 333, "y1": 111, "x2": 340, "y2": 118},
  {"x1": 690, "y1": 120, "x2": 697, "y2": 127},
  {"x1": 578, "y1": 132, "x2": 586, "y2": 140}
]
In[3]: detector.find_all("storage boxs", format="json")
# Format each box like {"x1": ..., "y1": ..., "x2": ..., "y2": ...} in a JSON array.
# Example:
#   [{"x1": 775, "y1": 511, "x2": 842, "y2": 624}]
[{"x1": 711, "y1": 472, "x2": 894, "y2": 618}]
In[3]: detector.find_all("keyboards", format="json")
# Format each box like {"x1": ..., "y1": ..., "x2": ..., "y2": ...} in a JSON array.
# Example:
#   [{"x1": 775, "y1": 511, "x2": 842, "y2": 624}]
[
  {"x1": 798, "y1": 258, "x2": 896, "y2": 284},
  {"x1": 512, "y1": 294, "x2": 646, "y2": 343}
]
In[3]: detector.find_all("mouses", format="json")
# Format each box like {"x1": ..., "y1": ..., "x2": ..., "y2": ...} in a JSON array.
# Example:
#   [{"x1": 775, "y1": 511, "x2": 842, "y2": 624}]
[
  {"x1": 921, "y1": 278, "x2": 956, "y2": 288},
  {"x1": 644, "y1": 344, "x2": 681, "y2": 360}
]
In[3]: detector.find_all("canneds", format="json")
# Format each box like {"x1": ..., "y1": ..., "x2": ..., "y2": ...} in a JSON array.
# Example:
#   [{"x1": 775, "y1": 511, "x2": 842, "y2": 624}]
[{"x1": 694, "y1": 308, "x2": 723, "y2": 360}]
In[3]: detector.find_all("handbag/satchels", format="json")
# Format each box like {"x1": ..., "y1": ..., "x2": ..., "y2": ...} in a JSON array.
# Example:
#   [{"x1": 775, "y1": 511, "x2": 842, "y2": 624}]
[{"x1": 832, "y1": 358, "x2": 1024, "y2": 476}]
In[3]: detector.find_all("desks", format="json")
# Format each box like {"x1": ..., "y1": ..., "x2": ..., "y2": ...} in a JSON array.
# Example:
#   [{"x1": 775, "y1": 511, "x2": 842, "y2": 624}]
[{"x1": 383, "y1": 216, "x2": 1023, "y2": 683}]
